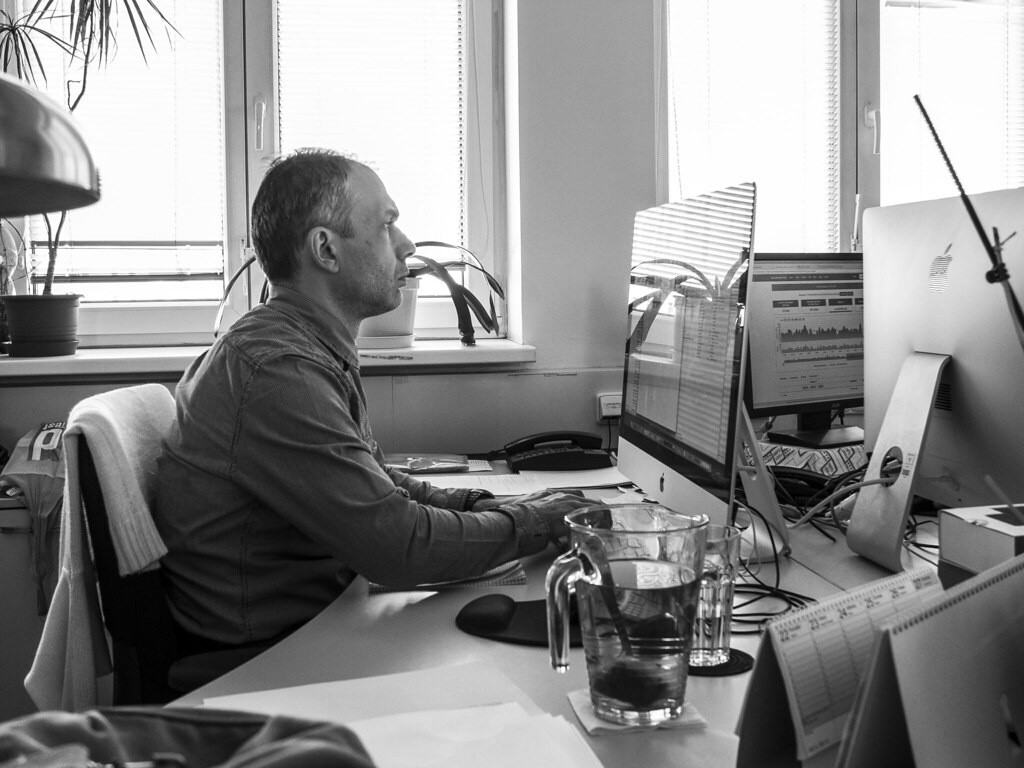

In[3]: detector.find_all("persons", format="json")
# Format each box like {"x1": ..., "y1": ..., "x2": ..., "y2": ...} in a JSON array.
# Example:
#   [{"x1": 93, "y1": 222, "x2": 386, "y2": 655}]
[{"x1": 109, "y1": 150, "x2": 612, "y2": 713}]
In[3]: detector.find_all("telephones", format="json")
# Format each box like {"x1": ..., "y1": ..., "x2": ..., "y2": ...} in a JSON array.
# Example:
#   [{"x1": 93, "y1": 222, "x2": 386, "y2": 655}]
[{"x1": 504, "y1": 429, "x2": 612, "y2": 474}]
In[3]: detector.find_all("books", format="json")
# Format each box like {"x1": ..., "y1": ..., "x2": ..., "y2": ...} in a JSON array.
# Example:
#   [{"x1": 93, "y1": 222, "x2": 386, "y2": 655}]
[{"x1": 369, "y1": 556, "x2": 526, "y2": 592}]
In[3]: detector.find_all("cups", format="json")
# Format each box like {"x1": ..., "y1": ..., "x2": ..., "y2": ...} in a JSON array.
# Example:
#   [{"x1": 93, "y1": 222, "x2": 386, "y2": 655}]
[{"x1": 689, "y1": 524, "x2": 741, "y2": 666}]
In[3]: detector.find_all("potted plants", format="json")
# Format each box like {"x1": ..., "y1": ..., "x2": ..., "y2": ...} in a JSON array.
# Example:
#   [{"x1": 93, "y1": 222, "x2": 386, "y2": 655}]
[
  {"x1": 214, "y1": 241, "x2": 505, "y2": 349},
  {"x1": 0, "y1": 0, "x2": 184, "y2": 359}
]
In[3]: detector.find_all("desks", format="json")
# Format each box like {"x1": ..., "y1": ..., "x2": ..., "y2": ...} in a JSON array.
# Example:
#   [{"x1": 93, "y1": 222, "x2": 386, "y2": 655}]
[{"x1": 164, "y1": 451, "x2": 943, "y2": 767}]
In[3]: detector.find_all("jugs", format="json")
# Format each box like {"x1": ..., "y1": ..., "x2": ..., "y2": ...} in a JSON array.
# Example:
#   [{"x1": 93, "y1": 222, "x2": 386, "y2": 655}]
[{"x1": 551, "y1": 503, "x2": 710, "y2": 728}]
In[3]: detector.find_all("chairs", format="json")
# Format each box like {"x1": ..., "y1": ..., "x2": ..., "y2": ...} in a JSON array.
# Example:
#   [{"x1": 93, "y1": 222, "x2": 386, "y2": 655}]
[{"x1": 22, "y1": 382, "x2": 301, "y2": 710}]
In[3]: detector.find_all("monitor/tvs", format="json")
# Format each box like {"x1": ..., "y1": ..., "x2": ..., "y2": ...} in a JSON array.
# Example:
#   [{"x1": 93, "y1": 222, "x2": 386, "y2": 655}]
[{"x1": 617, "y1": 182, "x2": 1024, "y2": 576}]
